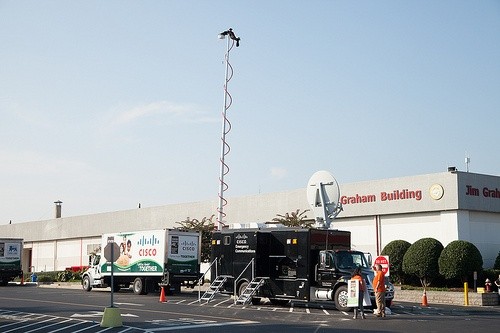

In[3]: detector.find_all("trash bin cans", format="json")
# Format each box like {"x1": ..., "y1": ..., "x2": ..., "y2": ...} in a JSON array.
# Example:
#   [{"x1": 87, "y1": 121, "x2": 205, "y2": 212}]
[{"x1": 31, "y1": 274, "x2": 37, "y2": 282}]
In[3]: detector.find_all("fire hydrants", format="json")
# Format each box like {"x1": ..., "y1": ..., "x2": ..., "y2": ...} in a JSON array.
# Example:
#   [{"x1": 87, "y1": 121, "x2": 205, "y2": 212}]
[{"x1": 484, "y1": 278, "x2": 492, "y2": 293}]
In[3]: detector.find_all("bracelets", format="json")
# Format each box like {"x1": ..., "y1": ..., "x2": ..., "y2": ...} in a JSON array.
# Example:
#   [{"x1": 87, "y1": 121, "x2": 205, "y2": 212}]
[{"x1": 376, "y1": 288, "x2": 378, "y2": 289}]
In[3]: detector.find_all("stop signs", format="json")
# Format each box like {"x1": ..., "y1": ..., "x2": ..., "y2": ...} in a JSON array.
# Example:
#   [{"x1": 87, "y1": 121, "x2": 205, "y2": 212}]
[{"x1": 374, "y1": 256, "x2": 389, "y2": 275}]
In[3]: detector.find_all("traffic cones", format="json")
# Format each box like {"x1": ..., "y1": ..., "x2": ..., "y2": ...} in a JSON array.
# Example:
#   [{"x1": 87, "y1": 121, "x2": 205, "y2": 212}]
[
  {"x1": 159, "y1": 286, "x2": 168, "y2": 302},
  {"x1": 420, "y1": 289, "x2": 430, "y2": 307}
]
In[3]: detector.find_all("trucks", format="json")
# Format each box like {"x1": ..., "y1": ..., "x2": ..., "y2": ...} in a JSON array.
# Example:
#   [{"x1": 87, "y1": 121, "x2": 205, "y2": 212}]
[
  {"x1": 81, "y1": 228, "x2": 205, "y2": 295},
  {"x1": 210, "y1": 226, "x2": 395, "y2": 312},
  {"x1": 0, "y1": 237, "x2": 24, "y2": 287}
]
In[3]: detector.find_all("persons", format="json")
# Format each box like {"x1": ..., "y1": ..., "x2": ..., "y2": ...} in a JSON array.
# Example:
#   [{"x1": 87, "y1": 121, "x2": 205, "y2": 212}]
[
  {"x1": 373, "y1": 265, "x2": 385, "y2": 318},
  {"x1": 351, "y1": 268, "x2": 366, "y2": 319},
  {"x1": 119, "y1": 243, "x2": 124, "y2": 253},
  {"x1": 124, "y1": 240, "x2": 132, "y2": 258}
]
